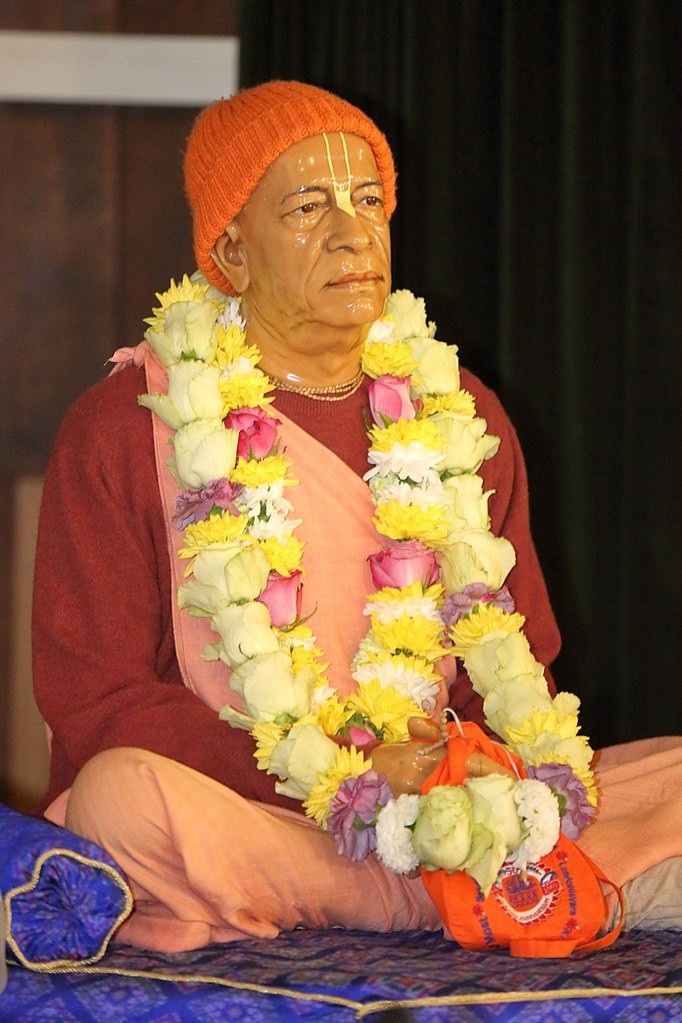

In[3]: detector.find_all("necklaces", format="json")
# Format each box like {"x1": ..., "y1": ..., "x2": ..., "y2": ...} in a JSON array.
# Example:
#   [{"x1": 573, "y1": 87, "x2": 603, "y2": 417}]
[
  {"x1": 268, "y1": 368, "x2": 365, "y2": 402},
  {"x1": 139, "y1": 273, "x2": 600, "y2": 902}
]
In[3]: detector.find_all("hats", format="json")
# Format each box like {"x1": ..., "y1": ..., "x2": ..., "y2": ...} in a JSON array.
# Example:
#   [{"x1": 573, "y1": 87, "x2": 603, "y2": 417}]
[{"x1": 182, "y1": 79, "x2": 399, "y2": 298}]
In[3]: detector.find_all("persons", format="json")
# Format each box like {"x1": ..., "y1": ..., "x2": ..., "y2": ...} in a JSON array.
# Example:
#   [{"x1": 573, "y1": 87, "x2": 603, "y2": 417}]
[{"x1": 30, "y1": 80, "x2": 681, "y2": 956}]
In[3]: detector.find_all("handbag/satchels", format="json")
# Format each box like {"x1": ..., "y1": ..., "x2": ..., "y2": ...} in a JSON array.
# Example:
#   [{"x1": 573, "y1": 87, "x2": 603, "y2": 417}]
[{"x1": 407, "y1": 713, "x2": 625, "y2": 961}]
